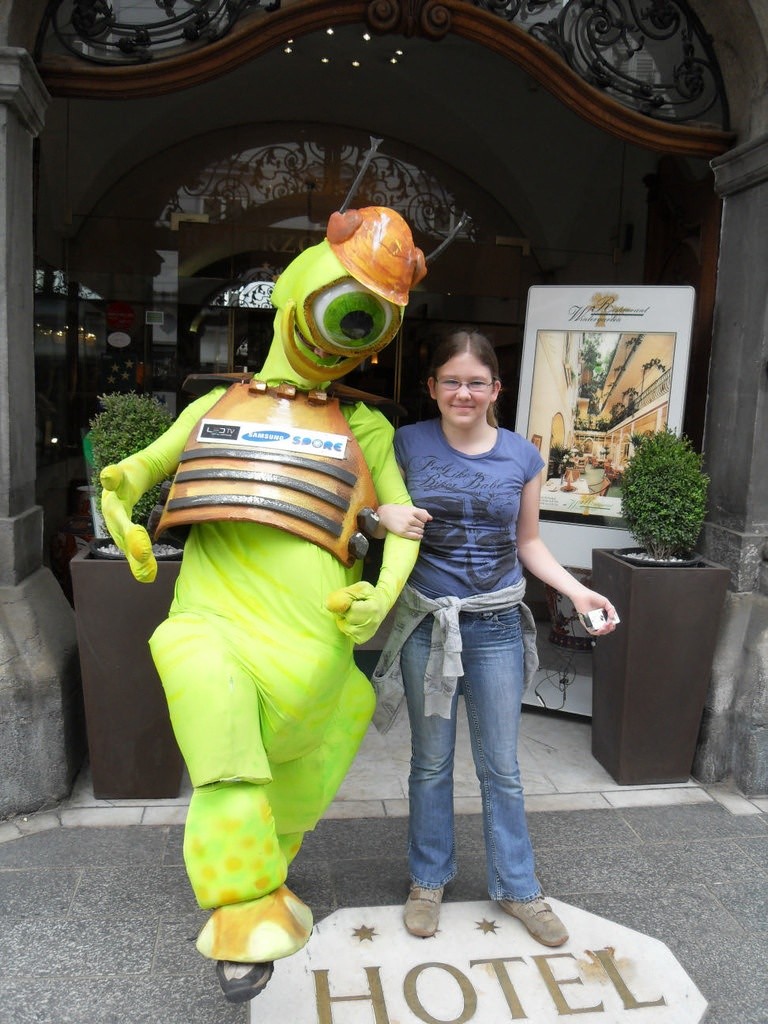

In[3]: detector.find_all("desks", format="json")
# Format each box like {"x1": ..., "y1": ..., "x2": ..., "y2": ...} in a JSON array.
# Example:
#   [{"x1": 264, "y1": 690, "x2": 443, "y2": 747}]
[
  {"x1": 611, "y1": 466, "x2": 624, "y2": 487},
  {"x1": 541, "y1": 478, "x2": 589, "y2": 495},
  {"x1": 597, "y1": 459, "x2": 608, "y2": 463}
]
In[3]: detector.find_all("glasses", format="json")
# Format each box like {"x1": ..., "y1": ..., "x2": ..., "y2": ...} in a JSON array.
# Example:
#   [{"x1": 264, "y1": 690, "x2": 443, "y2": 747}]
[{"x1": 434, "y1": 375, "x2": 495, "y2": 391}]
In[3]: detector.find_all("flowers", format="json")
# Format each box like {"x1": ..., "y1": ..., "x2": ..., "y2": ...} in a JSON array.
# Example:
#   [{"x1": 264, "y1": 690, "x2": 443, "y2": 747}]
[{"x1": 556, "y1": 448, "x2": 580, "y2": 473}]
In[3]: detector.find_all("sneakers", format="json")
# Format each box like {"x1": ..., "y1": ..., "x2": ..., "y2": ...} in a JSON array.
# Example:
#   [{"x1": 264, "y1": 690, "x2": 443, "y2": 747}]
[
  {"x1": 216, "y1": 959, "x2": 273, "y2": 1003},
  {"x1": 499, "y1": 895, "x2": 569, "y2": 947},
  {"x1": 403, "y1": 881, "x2": 444, "y2": 937}
]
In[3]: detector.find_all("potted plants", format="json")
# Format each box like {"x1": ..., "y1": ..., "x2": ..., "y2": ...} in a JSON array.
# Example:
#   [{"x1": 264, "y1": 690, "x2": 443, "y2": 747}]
[
  {"x1": 591, "y1": 422, "x2": 730, "y2": 786},
  {"x1": 69, "y1": 390, "x2": 184, "y2": 799}
]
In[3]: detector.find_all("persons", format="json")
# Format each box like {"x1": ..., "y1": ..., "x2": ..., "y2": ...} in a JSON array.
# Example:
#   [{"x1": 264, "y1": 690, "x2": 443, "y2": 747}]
[
  {"x1": 366, "y1": 332, "x2": 617, "y2": 950},
  {"x1": 98, "y1": 200, "x2": 429, "y2": 1000}
]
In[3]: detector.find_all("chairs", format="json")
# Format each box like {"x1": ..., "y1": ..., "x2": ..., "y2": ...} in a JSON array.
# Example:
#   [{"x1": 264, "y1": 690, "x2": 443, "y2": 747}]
[
  {"x1": 589, "y1": 476, "x2": 612, "y2": 497},
  {"x1": 576, "y1": 457, "x2": 588, "y2": 473},
  {"x1": 604, "y1": 463, "x2": 620, "y2": 487}
]
